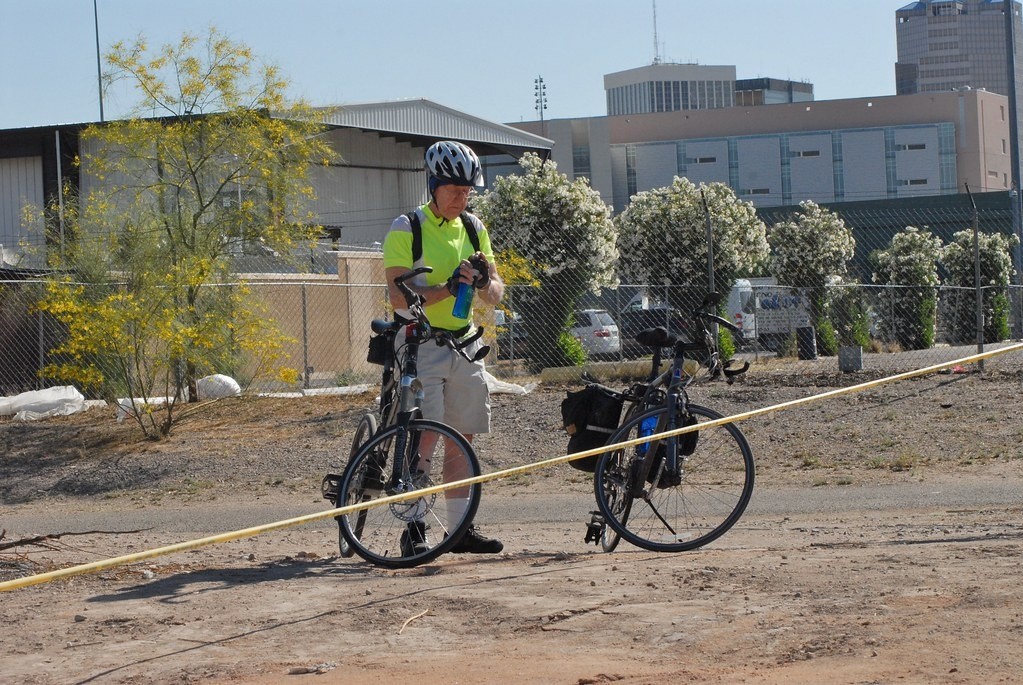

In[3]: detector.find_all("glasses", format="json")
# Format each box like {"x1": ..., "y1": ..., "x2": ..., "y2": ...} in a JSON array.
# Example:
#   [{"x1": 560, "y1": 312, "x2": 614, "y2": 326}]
[{"x1": 444, "y1": 186, "x2": 477, "y2": 200}]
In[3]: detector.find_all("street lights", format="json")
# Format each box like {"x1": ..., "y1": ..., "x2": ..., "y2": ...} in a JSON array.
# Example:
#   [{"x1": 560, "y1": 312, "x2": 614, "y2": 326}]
[{"x1": 534, "y1": 75, "x2": 548, "y2": 138}]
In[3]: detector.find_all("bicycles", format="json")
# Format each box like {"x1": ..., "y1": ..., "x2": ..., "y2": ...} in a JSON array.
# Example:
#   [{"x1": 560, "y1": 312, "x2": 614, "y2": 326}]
[
  {"x1": 584, "y1": 291, "x2": 756, "y2": 551},
  {"x1": 320, "y1": 266, "x2": 481, "y2": 568}
]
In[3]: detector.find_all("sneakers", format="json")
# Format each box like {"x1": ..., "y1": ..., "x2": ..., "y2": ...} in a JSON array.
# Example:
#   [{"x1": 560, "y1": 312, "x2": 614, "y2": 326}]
[
  {"x1": 399, "y1": 521, "x2": 435, "y2": 563},
  {"x1": 443, "y1": 524, "x2": 503, "y2": 553}
]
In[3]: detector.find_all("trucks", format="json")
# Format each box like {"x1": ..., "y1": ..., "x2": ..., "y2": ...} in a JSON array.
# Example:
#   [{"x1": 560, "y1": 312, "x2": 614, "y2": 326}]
[{"x1": 747, "y1": 276, "x2": 844, "y2": 351}]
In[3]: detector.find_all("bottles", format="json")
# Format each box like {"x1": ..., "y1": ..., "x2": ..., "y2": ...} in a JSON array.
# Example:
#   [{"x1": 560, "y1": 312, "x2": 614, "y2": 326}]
[
  {"x1": 639, "y1": 414, "x2": 659, "y2": 451},
  {"x1": 452, "y1": 253, "x2": 482, "y2": 319}
]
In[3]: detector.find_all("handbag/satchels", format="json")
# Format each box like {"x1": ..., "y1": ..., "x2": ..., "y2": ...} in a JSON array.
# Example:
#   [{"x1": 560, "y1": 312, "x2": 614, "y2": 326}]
[{"x1": 561, "y1": 383, "x2": 624, "y2": 474}]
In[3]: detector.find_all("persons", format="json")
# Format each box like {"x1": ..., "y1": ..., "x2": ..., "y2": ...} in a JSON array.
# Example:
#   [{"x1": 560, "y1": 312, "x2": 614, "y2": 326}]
[{"x1": 383, "y1": 140, "x2": 503, "y2": 558}]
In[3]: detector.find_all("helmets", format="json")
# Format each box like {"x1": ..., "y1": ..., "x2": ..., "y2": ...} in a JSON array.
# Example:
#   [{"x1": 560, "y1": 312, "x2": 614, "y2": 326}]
[{"x1": 424, "y1": 140, "x2": 482, "y2": 186}]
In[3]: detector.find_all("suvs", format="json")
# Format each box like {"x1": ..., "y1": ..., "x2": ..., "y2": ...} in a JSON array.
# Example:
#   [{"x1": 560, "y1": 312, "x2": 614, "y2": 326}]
[{"x1": 614, "y1": 309, "x2": 691, "y2": 353}]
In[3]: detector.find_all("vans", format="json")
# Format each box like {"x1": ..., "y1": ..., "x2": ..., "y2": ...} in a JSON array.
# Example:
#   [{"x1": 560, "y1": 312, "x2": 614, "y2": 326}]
[
  {"x1": 620, "y1": 279, "x2": 759, "y2": 352},
  {"x1": 499, "y1": 309, "x2": 622, "y2": 363}
]
